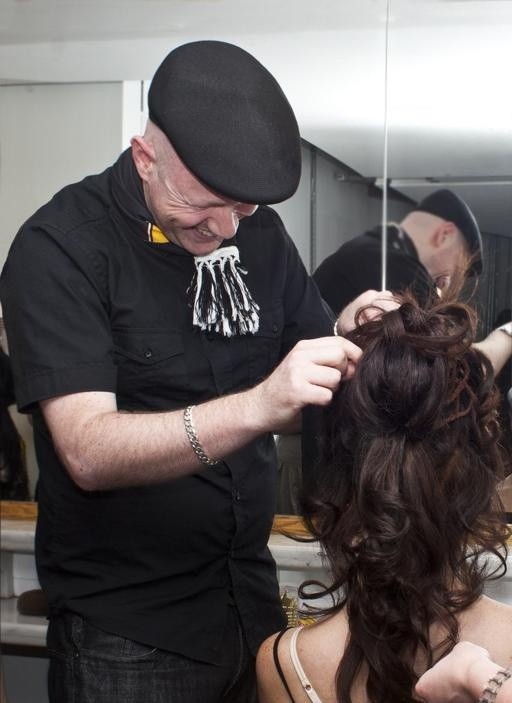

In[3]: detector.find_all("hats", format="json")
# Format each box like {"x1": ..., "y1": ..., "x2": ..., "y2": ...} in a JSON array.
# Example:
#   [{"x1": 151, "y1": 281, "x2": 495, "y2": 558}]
[
  {"x1": 148, "y1": 40, "x2": 302, "y2": 205},
  {"x1": 417, "y1": 188, "x2": 483, "y2": 278}
]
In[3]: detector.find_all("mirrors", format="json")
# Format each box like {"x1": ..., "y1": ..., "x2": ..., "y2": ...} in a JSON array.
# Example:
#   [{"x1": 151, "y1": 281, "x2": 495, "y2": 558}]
[
  {"x1": 0, "y1": 83, "x2": 122, "y2": 522},
  {"x1": 139, "y1": 77, "x2": 512, "y2": 547}
]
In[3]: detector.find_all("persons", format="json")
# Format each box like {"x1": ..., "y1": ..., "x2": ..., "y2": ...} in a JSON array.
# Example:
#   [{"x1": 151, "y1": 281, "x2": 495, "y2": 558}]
[
  {"x1": 297, "y1": 186, "x2": 510, "y2": 515},
  {"x1": 254, "y1": 305, "x2": 511, "y2": 703},
  {"x1": 0, "y1": 38, "x2": 358, "y2": 702},
  {"x1": 408, "y1": 640, "x2": 510, "y2": 703}
]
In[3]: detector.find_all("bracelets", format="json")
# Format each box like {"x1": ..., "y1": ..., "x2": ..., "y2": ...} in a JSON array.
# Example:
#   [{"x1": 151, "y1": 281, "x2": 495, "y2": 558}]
[
  {"x1": 183, "y1": 405, "x2": 221, "y2": 470},
  {"x1": 477, "y1": 668, "x2": 510, "y2": 703},
  {"x1": 498, "y1": 320, "x2": 512, "y2": 336}
]
